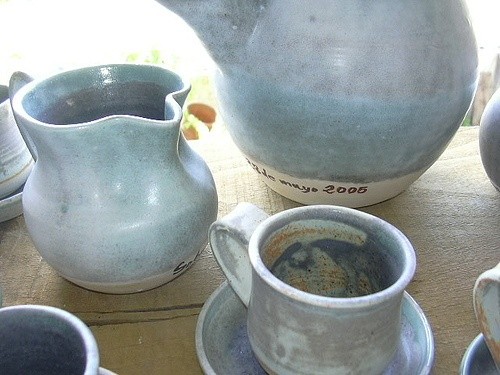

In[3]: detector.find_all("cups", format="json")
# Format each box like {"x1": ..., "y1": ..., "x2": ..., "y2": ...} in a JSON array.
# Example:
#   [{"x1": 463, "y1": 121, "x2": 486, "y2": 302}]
[
  {"x1": 209, "y1": 204, "x2": 417, "y2": 375},
  {"x1": 0, "y1": 305, "x2": 117, "y2": 375},
  {"x1": 474, "y1": 262, "x2": 500, "y2": 375},
  {"x1": 0, "y1": 84, "x2": 35, "y2": 199}
]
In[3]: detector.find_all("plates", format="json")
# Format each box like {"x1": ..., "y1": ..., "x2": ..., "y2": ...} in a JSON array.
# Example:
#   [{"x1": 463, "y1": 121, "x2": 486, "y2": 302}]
[
  {"x1": 460, "y1": 332, "x2": 500, "y2": 375},
  {"x1": 0, "y1": 190, "x2": 24, "y2": 223},
  {"x1": 195, "y1": 280, "x2": 436, "y2": 375}
]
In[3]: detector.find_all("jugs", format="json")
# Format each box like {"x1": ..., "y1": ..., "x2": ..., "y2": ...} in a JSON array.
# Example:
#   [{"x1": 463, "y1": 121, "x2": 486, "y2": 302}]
[
  {"x1": 157, "y1": 0, "x2": 479, "y2": 208},
  {"x1": 11, "y1": 64, "x2": 218, "y2": 294}
]
